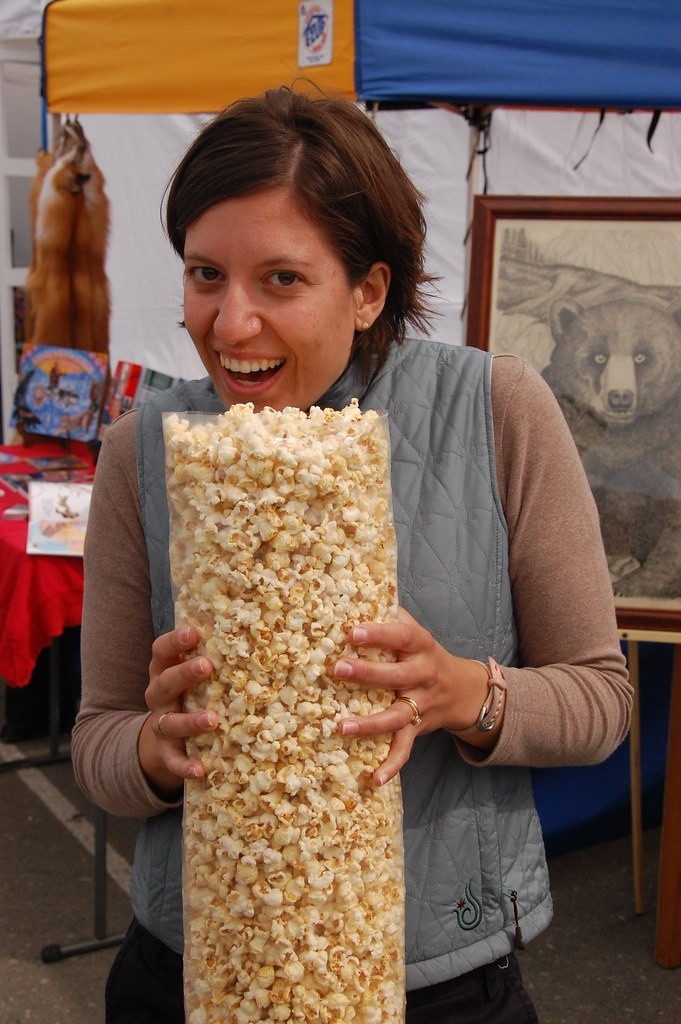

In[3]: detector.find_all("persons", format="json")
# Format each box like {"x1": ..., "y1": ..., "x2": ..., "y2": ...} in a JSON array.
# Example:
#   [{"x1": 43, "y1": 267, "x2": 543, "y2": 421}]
[{"x1": 70, "y1": 88, "x2": 633, "y2": 1024}]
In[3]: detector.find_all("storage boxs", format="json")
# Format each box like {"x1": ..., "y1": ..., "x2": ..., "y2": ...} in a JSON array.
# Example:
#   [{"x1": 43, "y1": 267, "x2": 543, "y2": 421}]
[{"x1": 16, "y1": 343, "x2": 183, "y2": 465}]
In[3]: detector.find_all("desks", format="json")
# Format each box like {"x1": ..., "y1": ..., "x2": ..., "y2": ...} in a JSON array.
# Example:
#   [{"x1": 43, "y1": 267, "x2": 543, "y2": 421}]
[{"x1": 0, "y1": 436, "x2": 128, "y2": 961}]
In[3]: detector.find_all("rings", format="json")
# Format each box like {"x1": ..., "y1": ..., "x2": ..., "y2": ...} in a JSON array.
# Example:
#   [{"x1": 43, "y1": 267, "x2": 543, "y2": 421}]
[
  {"x1": 158, "y1": 711, "x2": 177, "y2": 740},
  {"x1": 393, "y1": 696, "x2": 421, "y2": 727}
]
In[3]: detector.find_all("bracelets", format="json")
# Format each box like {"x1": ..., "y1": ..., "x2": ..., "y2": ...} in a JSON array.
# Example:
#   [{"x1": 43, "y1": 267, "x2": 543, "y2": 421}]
[{"x1": 449, "y1": 657, "x2": 507, "y2": 737}]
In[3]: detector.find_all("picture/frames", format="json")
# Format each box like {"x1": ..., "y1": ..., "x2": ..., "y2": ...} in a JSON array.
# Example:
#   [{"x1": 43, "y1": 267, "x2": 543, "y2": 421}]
[{"x1": 465, "y1": 193, "x2": 681, "y2": 638}]
